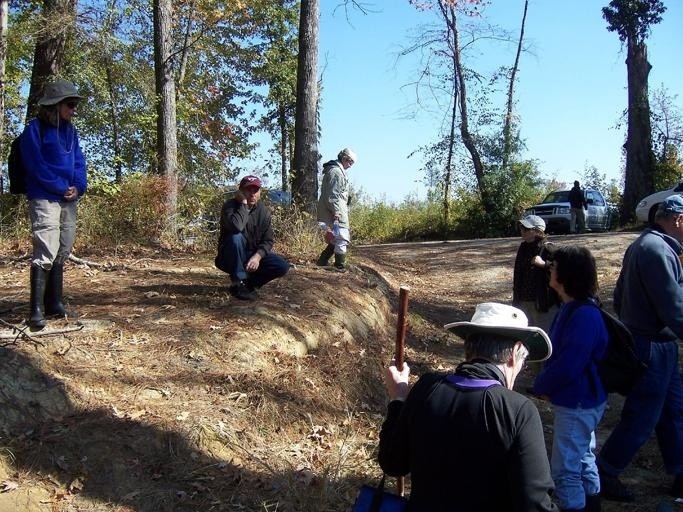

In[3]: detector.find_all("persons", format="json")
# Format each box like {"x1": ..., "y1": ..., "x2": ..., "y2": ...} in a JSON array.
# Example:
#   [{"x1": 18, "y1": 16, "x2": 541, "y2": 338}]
[
  {"x1": 314, "y1": 148, "x2": 357, "y2": 269},
  {"x1": 513, "y1": 213, "x2": 559, "y2": 377},
  {"x1": 20, "y1": 80, "x2": 87, "y2": 329},
  {"x1": 569, "y1": 181, "x2": 592, "y2": 233},
  {"x1": 213, "y1": 175, "x2": 288, "y2": 300},
  {"x1": 378, "y1": 300, "x2": 562, "y2": 512},
  {"x1": 595, "y1": 193, "x2": 683, "y2": 503},
  {"x1": 530, "y1": 244, "x2": 611, "y2": 512}
]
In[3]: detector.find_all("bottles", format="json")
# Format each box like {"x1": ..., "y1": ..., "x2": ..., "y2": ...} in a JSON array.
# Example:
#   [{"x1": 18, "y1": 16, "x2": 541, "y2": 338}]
[
  {"x1": 656, "y1": 499, "x2": 673, "y2": 512},
  {"x1": 332, "y1": 217, "x2": 340, "y2": 237}
]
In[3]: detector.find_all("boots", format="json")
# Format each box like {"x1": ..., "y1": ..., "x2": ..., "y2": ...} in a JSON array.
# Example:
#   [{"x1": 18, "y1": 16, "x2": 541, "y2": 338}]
[
  {"x1": 46, "y1": 264, "x2": 77, "y2": 319},
  {"x1": 316, "y1": 243, "x2": 335, "y2": 266},
  {"x1": 30, "y1": 270, "x2": 50, "y2": 327},
  {"x1": 335, "y1": 254, "x2": 350, "y2": 268}
]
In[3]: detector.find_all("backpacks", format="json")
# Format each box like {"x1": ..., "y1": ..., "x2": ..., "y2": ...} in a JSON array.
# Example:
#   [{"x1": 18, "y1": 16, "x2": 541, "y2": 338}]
[
  {"x1": 8, "y1": 113, "x2": 45, "y2": 194},
  {"x1": 549, "y1": 296, "x2": 648, "y2": 397}
]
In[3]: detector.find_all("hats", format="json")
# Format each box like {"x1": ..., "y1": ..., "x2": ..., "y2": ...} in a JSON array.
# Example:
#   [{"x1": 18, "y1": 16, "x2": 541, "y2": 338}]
[
  {"x1": 343, "y1": 147, "x2": 357, "y2": 163},
  {"x1": 517, "y1": 214, "x2": 547, "y2": 232},
  {"x1": 660, "y1": 194, "x2": 683, "y2": 214},
  {"x1": 444, "y1": 302, "x2": 553, "y2": 363},
  {"x1": 39, "y1": 81, "x2": 88, "y2": 106},
  {"x1": 240, "y1": 176, "x2": 261, "y2": 188}
]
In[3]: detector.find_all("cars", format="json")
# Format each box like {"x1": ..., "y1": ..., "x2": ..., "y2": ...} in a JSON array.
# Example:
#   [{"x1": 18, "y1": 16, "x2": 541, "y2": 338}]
[{"x1": 635, "y1": 181, "x2": 682, "y2": 227}]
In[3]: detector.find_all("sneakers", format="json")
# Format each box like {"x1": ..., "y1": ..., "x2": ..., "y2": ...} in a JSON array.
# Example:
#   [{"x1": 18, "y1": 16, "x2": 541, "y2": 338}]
[
  {"x1": 240, "y1": 279, "x2": 257, "y2": 295},
  {"x1": 228, "y1": 284, "x2": 251, "y2": 300},
  {"x1": 599, "y1": 479, "x2": 635, "y2": 502}
]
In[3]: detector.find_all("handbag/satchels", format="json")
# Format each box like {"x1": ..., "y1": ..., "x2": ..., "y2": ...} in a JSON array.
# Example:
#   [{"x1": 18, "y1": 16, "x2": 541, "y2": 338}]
[{"x1": 351, "y1": 472, "x2": 408, "y2": 512}]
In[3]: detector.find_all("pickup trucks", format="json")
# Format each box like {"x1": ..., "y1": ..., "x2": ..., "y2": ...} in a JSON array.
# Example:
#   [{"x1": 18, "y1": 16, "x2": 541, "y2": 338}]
[{"x1": 524, "y1": 188, "x2": 620, "y2": 235}]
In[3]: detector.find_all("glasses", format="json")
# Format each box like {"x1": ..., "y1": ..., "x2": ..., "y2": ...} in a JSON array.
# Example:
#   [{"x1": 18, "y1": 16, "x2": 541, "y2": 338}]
[
  {"x1": 62, "y1": 101, "x2": 80, "y2": 109},
  {"x1": 519, "y1": 225, "x2": 538, "y2": 232}
]
